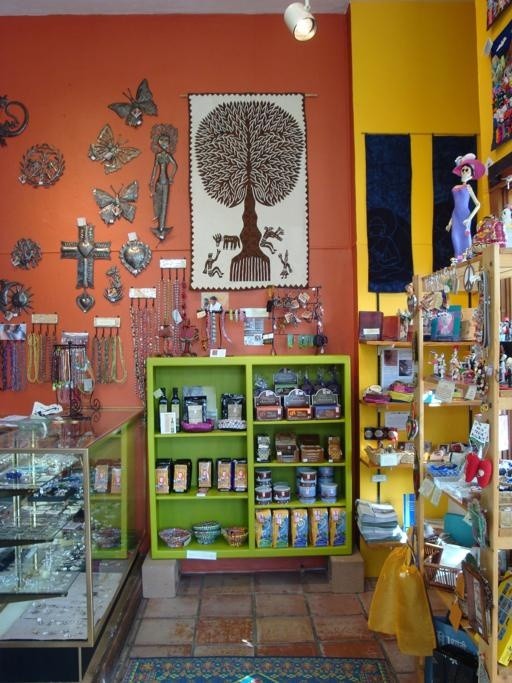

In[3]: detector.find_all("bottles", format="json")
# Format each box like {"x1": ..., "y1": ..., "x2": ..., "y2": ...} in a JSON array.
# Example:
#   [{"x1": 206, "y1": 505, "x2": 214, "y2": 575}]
[
  {"x1": 158, "y1": 387, "x2": 168, "y2": 432},
  {"x1": 170, "y1": 386, "x2": 181, "y2": 432}
]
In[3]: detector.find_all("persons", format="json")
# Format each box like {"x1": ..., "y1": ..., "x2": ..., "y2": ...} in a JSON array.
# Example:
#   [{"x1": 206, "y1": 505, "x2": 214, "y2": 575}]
[
  {"x1": 209, "y1": 295, "x2": 223, "y2": 311},
  {"x1": 148, "y1": 123, "x2": 179, "y2": 241},
  {"x1": 445, "y1": 152, "x2": 487, "y2": 258},
  {"x1": 202, "y1": 296, "x2": 208, "y2": 312}
]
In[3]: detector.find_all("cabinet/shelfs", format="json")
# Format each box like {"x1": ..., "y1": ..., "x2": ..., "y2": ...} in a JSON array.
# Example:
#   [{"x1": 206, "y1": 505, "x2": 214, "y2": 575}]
[
  {"x1": 413, "y1": 242, "x2": 512, "y2": 683},
  {"x1": 0, "y1": 407, "x2": 143, "y2": 646},
  {"x1": 146, "y1": 354, "x2": 352, "y2": 559},
  {"x1": 360, "y1": 337, "x2": 481, "y2": 550},
  {"x1": 284, "y1": 0, "x2": 317, "y2": 41}
]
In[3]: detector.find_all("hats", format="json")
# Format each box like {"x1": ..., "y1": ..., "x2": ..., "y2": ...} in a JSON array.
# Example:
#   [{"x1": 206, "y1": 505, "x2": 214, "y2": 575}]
[{"x1": 452, "y1": 154, "x2": 485, "y2": 180}]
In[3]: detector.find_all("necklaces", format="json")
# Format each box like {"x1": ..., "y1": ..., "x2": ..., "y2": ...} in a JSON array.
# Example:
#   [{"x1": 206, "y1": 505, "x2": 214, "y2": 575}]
[
  {"x1": 0, "y1": 332, "x2": 127, "y2": 401},
  {"x1": 128, "y1": 303, "x2": 156, "y2": 405},
  {"x1": 196, "y1": 311, "x2": 239, "y2": 356},
  {"x1": 156, "y1": 281, "x2": 190, "y2": 356}
]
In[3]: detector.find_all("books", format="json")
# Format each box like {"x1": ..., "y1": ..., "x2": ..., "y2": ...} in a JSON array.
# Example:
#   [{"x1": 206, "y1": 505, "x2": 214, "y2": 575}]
[
  {"x1": 353, "y1": 498, "x2": 404, "y2": 544},
  {"x1": 474, "y1": 567, "x2": 512, "y2": 668}
]
